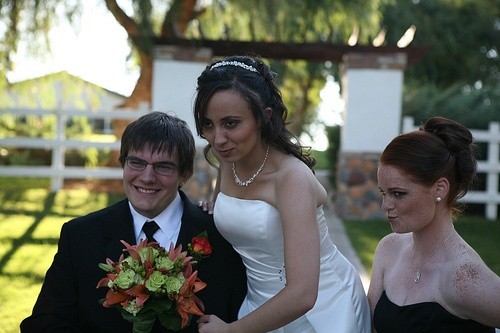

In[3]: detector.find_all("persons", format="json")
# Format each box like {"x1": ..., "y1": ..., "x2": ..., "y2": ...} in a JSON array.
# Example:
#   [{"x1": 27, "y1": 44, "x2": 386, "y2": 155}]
[
  {"x1": 366, "y1": 118, "x2": 500, "y2": 333},
  {"x1": 194, "y1": 55, "x2": 371, "y2": 333},
  {"x1": 20, "y1": 111, "x2": 248, "y2": 333}
]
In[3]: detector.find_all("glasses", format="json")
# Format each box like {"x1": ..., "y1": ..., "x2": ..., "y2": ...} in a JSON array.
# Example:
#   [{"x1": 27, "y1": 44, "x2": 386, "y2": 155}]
[{"x1": 124, "y1": 155, "x2": 179, "y2": 176}]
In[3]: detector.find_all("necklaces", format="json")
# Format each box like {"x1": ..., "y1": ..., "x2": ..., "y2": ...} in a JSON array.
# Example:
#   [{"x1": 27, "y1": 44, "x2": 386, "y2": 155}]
[
  {"x1": 414, "y1": 272, "x2": 421, "y2": 282},
  {"x1": 232, "y1": 144, "x2": 269, "y2": 187}
]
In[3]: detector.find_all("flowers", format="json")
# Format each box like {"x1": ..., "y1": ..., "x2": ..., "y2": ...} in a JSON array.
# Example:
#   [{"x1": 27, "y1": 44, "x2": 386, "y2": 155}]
[
  {"x1": 96, "y1": 239, "x2": 207, "y2": 333},
  {"x1": 187, "y1": 231, "x2": 212, "y2": 264}
]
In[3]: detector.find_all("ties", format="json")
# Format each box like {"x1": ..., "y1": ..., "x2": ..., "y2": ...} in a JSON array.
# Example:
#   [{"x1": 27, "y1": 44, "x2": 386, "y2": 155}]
[{"x1": 142, "y1": 219, "x2": 165, "y2": 248}]
